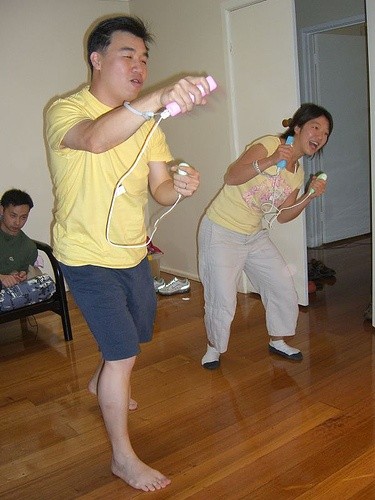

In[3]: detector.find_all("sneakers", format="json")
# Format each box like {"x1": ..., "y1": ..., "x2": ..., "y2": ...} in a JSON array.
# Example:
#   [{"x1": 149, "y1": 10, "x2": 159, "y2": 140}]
[
  {"x1": 159, "y1": 277, "x2": 191, "y2": 296},
  {"x1": 153, "y1": 278, "x2": 166, "y2": 293}
]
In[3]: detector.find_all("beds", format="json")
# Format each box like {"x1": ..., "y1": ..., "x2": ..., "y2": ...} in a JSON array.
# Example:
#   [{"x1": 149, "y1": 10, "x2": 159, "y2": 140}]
[{"x1": 0, "y1": 239, "x2": 74, "y2": 342}]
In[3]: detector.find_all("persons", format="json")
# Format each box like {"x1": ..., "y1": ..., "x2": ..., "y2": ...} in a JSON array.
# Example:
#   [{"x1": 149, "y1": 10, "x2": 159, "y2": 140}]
[
  {"x1": 196, "y1": 104, "x2": 333, "y2": 369},
  {"x1": 0, "y1": 188, "x2": 38, "y2": 288},
  {"x1": 43, "y1": 15, "x2": 212, "y2": 492}
]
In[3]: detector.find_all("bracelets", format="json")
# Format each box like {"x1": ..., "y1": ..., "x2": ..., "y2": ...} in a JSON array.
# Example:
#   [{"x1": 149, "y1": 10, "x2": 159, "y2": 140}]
[{"x1": 255, "y1": 159, "x2": 263, "y2": 173}]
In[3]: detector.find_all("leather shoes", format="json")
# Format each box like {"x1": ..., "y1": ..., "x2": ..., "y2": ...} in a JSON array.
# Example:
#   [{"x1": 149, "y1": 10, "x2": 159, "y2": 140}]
[
  {"x1": 308, "y1": 264, "x2": 321, "y2": 280},
  {"x1": 312, "y1": 259, "x2": 336, "y2": 276}
]
